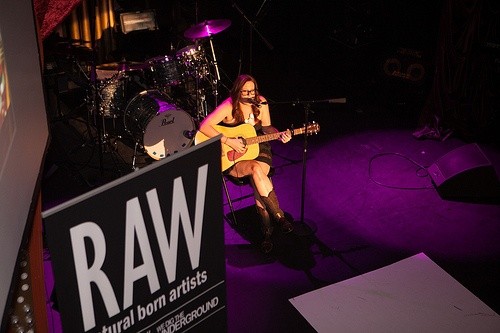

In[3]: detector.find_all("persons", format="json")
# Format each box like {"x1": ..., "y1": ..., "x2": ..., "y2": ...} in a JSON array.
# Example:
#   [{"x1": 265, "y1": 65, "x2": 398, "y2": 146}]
[{"x1": 199, "y1": 74, "x2": 293, "y2": 254}]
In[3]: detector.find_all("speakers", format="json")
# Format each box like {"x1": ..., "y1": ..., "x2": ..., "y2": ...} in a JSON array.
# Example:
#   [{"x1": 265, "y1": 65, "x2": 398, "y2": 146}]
[{"x1": 427, "y1": 142, "x2": 497, "y2": 200}]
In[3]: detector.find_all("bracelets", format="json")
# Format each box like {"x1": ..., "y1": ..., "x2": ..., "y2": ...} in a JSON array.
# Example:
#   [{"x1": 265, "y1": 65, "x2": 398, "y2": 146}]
[{"x1": 224, "y1": 137, "x2": 229, "y2": 144}]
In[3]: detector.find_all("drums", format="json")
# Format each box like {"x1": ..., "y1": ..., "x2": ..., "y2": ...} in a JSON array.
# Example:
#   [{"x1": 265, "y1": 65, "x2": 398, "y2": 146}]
[
  {"x1": 85, "y1": 72, "x2": 122, "y2": 119},
  {"x1": 175, "y1": 44, "x2": 209, "y2": 83},
  {"x1": 123, "y1": 89, "x2": 196, "y2": 161},
  {"x1": 147, "y1": 55, "x2": 182, "y2": 88}
]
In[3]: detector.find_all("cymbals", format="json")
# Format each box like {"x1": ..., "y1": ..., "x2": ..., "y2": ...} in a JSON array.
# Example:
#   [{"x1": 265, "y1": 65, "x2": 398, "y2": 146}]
[
  {"x1": 183, "y1": 19, "x2": 232, "y2": 39},
  {"x1": 96, "y1": 61, "x2": 147, "y2": 71}
]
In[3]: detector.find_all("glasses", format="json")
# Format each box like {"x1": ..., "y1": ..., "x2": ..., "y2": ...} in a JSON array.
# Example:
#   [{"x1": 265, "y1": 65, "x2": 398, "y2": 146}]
[{"x1": 241, "y1": 89, "x2": 255, "y2": 96}]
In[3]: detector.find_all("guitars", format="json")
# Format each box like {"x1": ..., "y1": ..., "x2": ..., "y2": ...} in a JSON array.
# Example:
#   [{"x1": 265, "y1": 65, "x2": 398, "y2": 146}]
[{"x1": 194, "y1": 121, "x2": 321, "y2": 173}]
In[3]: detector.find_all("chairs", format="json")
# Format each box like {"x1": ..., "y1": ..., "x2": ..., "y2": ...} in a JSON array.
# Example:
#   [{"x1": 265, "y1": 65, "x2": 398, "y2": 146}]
[{"x1": 221, "y1": 162, "x2": 275, "y2": 227}]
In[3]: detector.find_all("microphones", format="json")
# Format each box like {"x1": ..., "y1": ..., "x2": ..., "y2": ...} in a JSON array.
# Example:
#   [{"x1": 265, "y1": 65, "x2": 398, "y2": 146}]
[{"x1": 248, "y1": 98, "x2": 261, "y2": 108}]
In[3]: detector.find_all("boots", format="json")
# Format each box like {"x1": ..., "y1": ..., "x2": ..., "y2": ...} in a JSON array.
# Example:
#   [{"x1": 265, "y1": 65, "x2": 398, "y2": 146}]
[
  {"x1": 257, "y1": 205, "x2": 274, "y2": 238},
  {"x1": 260, "y1": 189, "x2": 293, "y2": 233}
]
